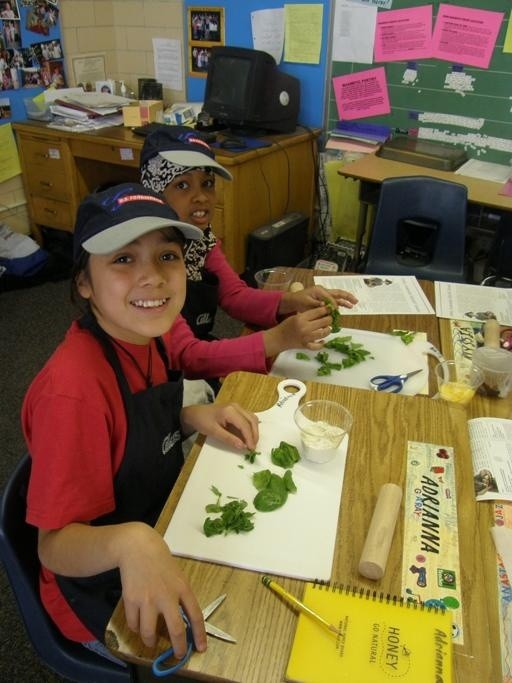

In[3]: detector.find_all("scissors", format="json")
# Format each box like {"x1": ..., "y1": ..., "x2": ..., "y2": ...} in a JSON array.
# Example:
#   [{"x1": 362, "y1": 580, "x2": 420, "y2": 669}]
[
  {"x1": 151, "y1": 594, "x2": 236, "y2": 677},
  {"x1": 370, "y1": 369, "x2": 424, "y2": 393}
]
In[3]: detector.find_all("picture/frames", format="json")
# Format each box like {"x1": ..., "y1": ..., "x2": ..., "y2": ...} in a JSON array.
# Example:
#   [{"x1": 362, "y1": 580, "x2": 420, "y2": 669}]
[{"x1": 186, "y1": 5, "x2": 226, "y2": 78}]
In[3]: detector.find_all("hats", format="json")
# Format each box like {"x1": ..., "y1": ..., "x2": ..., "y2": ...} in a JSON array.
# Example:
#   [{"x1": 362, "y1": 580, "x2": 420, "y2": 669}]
[
  {"x1": 139, "y1": 125, "x2": 234, "y2": 183},
  {"x1": 73, "y1": 182, "x2": 204, "y2": 256}
]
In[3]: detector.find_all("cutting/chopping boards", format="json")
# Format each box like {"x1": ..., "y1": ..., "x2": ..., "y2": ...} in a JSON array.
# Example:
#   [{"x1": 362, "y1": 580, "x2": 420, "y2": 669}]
[
  {"x1": 164, "y1": 379, "x2": 350, "y2": 583},
  {"x1": 264, "y1": 325, "x2": 448, "y2": 405}
]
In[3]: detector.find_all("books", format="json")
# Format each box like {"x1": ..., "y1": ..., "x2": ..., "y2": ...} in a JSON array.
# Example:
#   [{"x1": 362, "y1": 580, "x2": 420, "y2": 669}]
[
  {"x1": 53, "y1": 90, "x2": 140, "y2": 122},
  {"x1": 285, "y1": 580, "x2": 453, "y2": 682}
]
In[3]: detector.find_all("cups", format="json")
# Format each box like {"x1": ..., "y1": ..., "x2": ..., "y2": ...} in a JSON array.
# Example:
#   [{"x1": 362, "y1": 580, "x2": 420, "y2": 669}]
[
  {"x1": 433, "y1": 346, "x2": 512, "y2": 407},
  {"x1": 254, "y1": 268, "x2": 293, "y2": 290},
  {"x1": 296, "y1": 398, "x2": 355, "y2": 465}
]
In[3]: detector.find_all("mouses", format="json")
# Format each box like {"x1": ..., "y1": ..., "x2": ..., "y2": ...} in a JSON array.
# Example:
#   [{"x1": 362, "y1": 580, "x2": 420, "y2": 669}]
[{"x1": 220, "y1": 139, "x2": 248, "y2": 148}]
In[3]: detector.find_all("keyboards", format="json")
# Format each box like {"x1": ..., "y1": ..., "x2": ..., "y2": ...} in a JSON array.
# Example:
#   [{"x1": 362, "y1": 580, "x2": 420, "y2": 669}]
[{"x1": 131, "y1": 122, "x2": 217, "y2": 144}]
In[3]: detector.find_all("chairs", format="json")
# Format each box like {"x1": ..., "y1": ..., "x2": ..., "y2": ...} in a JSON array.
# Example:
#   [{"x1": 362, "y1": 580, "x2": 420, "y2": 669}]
[
  {"x1": 3, "y1": 453, "x2": 147, "y2": 682},
  {"x1": 362, "y1": 172, "x2": 472, "y2": 275}
]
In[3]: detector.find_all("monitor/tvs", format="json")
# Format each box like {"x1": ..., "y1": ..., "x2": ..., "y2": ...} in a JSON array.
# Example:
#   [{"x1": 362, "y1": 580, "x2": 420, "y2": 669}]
[{"x1": 203, "y1": 46, "x2": 300, "y2": 133}]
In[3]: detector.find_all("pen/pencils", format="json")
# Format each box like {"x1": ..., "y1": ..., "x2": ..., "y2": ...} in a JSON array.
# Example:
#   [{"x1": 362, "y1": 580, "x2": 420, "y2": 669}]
[{"x1": 261, "y1": 575, "x2": 344, "y2": 637}]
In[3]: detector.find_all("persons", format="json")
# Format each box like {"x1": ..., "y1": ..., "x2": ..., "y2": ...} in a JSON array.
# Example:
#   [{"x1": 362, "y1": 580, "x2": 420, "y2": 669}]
[
  {"x1": 0, "y1": 0, "x2": 66, "y2": 90},
  {"x1": 137, "y1": 125, "x2": 357, "y2": 393},
  {"x1": 20, "y1": 184, "x2": 259, "y2": 658},
  {"x1": 191, "y1": 12, "x2": 219, "y2": 70},
  {"x1": 465, "y1": 311, "x2": 495, "y2": 321},
  {"x1": 364, "y1": 278, "x2": 392, "y2": 287}
]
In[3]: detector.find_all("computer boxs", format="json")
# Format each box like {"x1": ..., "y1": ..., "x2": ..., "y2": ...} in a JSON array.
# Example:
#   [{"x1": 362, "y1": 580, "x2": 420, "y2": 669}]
[{"x1": 246, "y1": 212, "x2": 309, "y2": 278}]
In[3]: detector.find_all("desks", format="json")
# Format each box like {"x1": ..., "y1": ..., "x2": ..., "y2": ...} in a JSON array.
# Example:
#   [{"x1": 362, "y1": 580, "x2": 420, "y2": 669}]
[
  {"x1": 338, "y1": 150, "x2": 510, "y2": 276},
  {"x1": 106, "y1": 267, "x2": 511, "y2": 682},
  {"x1": 11, "y1": 102, "x2": 326, "y2": 269}
]
